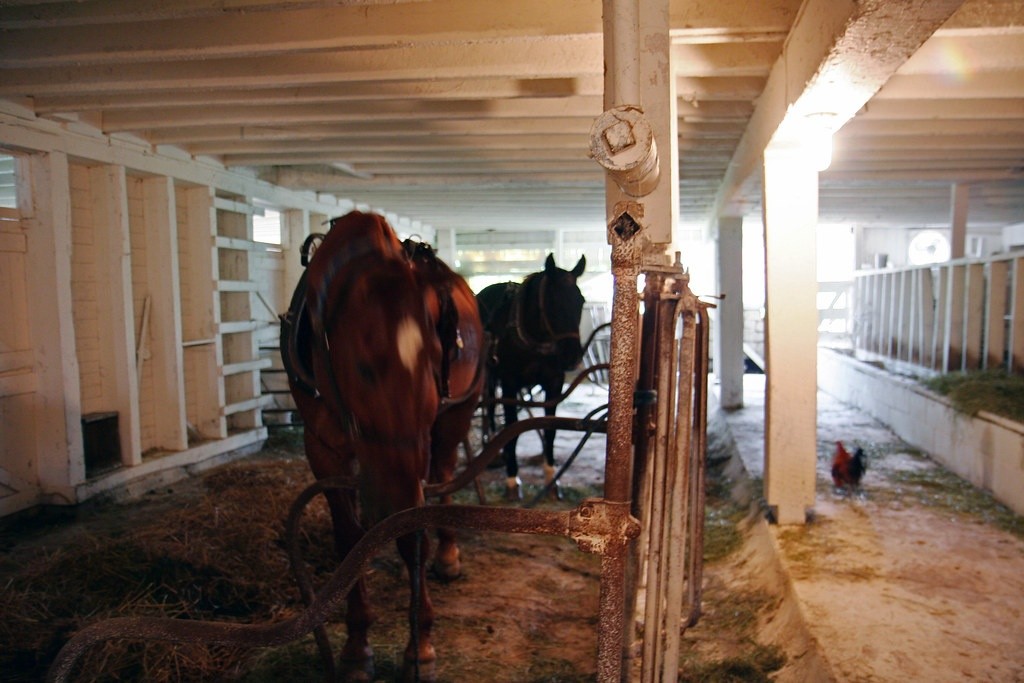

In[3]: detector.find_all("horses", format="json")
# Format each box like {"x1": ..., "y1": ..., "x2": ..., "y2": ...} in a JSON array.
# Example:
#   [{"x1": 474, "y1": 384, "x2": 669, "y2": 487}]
[{"x1": 279, "y1": 210, "x2": 586, "y2": 681}]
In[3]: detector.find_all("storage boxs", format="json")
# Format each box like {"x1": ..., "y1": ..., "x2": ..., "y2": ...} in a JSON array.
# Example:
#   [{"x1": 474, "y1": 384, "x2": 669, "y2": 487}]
[{"x1": 80, "y1": 410, "x2": 123, "y2": 478}]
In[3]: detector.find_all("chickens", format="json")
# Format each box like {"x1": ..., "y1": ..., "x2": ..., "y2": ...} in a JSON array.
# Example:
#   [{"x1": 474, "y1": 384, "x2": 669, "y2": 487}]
[{"x1": 830, "y1": 439, "x2": 866, "y2": 500}]
